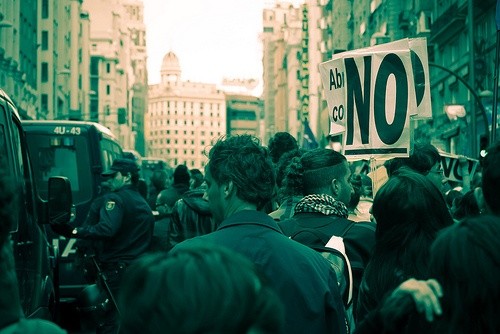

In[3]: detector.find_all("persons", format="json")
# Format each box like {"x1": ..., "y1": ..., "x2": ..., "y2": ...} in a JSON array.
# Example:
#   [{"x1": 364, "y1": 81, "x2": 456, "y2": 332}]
[
  {"x1": 120, "y1": 132, "x2": 500, "y2": 334},
  {"x1": 50, "y1": 158, "x2": 155, "y2": 334}
]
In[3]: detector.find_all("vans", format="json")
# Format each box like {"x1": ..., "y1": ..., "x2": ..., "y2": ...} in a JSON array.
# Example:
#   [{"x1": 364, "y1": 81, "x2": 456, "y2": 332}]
[
  {"x1": 0, "y1": 87, "x2": 72, "y2": 330},
  {"x1": 17, "y1": 120, "x2": 128, "y2": 307}
]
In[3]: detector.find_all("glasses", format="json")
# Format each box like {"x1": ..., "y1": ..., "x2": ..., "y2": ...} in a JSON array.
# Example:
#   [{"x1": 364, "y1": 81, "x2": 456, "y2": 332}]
[{"x1": 424, "y1": 168, "x2": 444, "y2": 176}]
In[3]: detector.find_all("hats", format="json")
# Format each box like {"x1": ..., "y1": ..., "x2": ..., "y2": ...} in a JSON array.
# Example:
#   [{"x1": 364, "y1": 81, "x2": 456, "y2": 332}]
[{"x1": 100, "y1": 157, "x2": 138, "y2": 175}]
